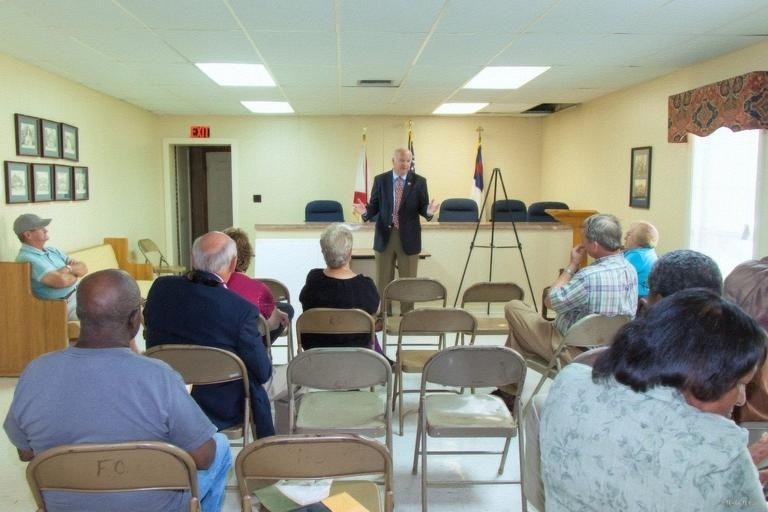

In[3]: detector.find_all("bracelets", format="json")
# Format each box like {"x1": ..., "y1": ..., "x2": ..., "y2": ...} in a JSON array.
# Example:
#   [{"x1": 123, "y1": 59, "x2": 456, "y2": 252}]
[{"x1": 564, "y1": 269, "x2": 573, "y2": 274}]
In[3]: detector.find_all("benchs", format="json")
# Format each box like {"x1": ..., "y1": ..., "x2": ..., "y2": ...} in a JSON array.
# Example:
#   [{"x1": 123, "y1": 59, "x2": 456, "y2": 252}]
[{"x1": 66, "y1": 244, "x2": 153, "y2": 341}]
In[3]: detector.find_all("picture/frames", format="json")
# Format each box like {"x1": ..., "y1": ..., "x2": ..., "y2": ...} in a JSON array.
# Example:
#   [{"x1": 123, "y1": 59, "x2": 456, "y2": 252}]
[
  {"x1": 629, "y1": 146, "x2": 651, "y2": 208},
  {"x1": 5, "y1": 114, "x2": 89, "y2": 205}
]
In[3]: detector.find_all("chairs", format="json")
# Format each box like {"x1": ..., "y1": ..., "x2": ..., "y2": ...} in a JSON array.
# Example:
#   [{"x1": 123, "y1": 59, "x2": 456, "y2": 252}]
[
  {"x1": 439, "y1": 198, "x2": 478, "y2": 222},
  {"x1": 528, "y1": 202, "x2": 569, "y2": 222},
  {"x1": 138, "y1": 239, "x2": 186, "y2": 277},
  {"x1": 491, "y1": 200, "x2": 527, "y2": 222},
  {"x1": 306, "y1": 200, "x2": 344, "y2": 222}
]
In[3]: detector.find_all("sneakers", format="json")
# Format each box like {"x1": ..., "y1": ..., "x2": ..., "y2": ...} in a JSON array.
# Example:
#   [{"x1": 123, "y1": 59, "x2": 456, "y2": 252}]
[{"x1": 489, "y1": 389, "x2": 517, "y2": 412}]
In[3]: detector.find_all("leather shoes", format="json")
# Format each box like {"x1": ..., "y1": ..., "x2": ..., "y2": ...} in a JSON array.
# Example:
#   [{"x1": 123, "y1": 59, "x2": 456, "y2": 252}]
[{"x1": 374, "y1": 316, "x2": 384, "y2": 332}]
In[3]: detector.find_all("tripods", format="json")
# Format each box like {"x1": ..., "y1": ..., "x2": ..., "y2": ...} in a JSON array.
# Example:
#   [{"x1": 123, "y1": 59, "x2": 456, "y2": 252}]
[{"x1": 453, "y1": 167, "x2": 540, "y2": 315}]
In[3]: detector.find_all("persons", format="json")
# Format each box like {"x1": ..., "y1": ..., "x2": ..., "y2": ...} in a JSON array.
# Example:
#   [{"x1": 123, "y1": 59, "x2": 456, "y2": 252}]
[
  {"x1": 222, "y1": 228, "x2": 294, "y2": 346},
  {"x1": 622, "y1": 221, "x2": 657, "y2": 298},
  {"x1": 299, "y1": 223, "x2": 394, "y2": 366},
  {"x1": 491, "y1": 214, "x2": 639, "y2": 417},
  {"x1": 352, "y1": 147, "x2": 439, "y2": 332},
  {"x1": 142, "y1": 231, "x2": 275, "y2": 439},
  {"x1": 540, "y1": 288, "x2": 767, "y2": 512},
  {"x1": 14, "y1": 213, "x2": 146, "y2": 352},
  {"x1": 647, "y1": 250, "x2": 766, "y2": 423},
  {"x1": 2, "y1": 268, "x2": 233, "y2": 511}
]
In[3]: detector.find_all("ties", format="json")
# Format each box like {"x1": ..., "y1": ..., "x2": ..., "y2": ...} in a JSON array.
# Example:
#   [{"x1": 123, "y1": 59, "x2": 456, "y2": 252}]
[{"x1": 392, "y1": 177, "x2": 403, "y2": 230}]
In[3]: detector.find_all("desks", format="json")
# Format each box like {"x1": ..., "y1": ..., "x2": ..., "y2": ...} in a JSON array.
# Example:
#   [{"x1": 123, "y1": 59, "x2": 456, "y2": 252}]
[{"x1": 253, "y1": 222, "x2": 574, "y2": 316}]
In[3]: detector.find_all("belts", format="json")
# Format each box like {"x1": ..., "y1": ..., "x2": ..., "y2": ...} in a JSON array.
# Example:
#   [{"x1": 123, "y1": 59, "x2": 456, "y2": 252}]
[
  {"x1": 576, "y1": 346, "x2": 590, "y2": 352},
  {"x1": 63, "y1": 285, "x2": 77, "y2": 299}
]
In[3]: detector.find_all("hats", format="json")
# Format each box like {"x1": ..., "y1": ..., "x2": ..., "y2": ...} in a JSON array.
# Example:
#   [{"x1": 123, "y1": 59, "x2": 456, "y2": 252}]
[
  {"x1": 251, "y1": 479, "x2": 332, "y2": 512},
  {"x1": 13, "y1": 213, "x2": 52, "y2": 237}
]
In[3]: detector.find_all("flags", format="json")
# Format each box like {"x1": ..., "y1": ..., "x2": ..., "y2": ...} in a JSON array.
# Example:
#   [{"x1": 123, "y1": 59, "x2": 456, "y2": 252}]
[
  {"x1": 351, "y1": 129, "x2": 373, "y2": 217},
  {"x1": 408, "y1": 121, "x2": 415, "y2": 174},
  {"x1": 474, "y1": 130, "x2": 483, "y2": 192}
]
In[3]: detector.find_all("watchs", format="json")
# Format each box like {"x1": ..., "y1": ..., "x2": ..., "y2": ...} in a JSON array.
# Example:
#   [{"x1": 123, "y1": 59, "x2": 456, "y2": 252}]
[{"x1": 66, "y1": 265, "x2": 72, "y2": 273}]
